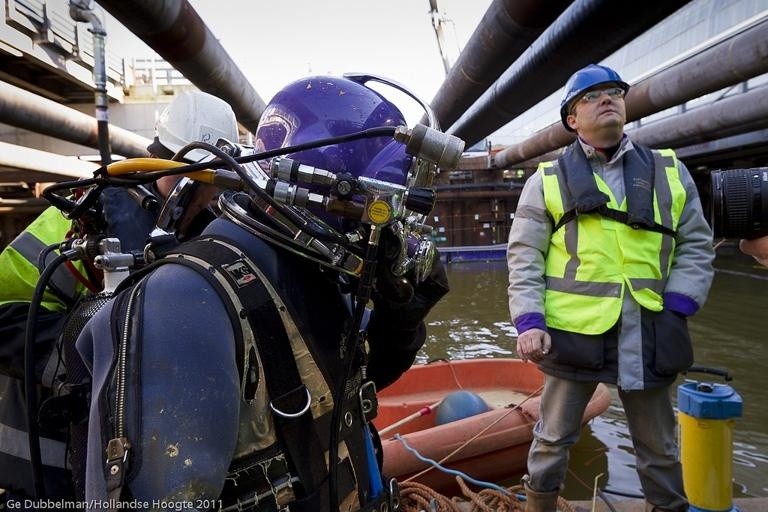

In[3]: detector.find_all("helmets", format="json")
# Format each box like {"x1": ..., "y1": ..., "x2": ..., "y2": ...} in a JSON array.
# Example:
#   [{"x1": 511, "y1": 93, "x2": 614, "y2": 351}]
[
  {"x1": 147, "y1": 89, "x2": 239, "y2": 168},
  {"x1": 253, "y1": 77, "x2": 414, "y2": 229},
  {"x1": 560, "y1": 64, "x2": 629, "y2": 132}
]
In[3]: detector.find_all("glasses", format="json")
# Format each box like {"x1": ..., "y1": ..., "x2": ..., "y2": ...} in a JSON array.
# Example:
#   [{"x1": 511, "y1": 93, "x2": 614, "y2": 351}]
[{"x1": 568, "y1": 87, "x2": 626, "y2": 115}]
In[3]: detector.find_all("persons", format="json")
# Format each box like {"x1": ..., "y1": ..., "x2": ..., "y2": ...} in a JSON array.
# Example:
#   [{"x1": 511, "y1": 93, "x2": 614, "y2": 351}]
[
  {"x1": 64, "y1": 70, "x2": 452, "y2": 512},
  {"x1": 505, "y1": 60, "x2": 716, "y2": 511},
  {"x1": 0, "y1": 87, "x2": 238, "y2": 509}
]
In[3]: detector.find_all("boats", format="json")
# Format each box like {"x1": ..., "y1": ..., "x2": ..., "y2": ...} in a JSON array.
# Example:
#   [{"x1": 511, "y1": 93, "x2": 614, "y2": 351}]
[{"x1": 369, "y1": 356, "x2": 611, "y2": 494}]
